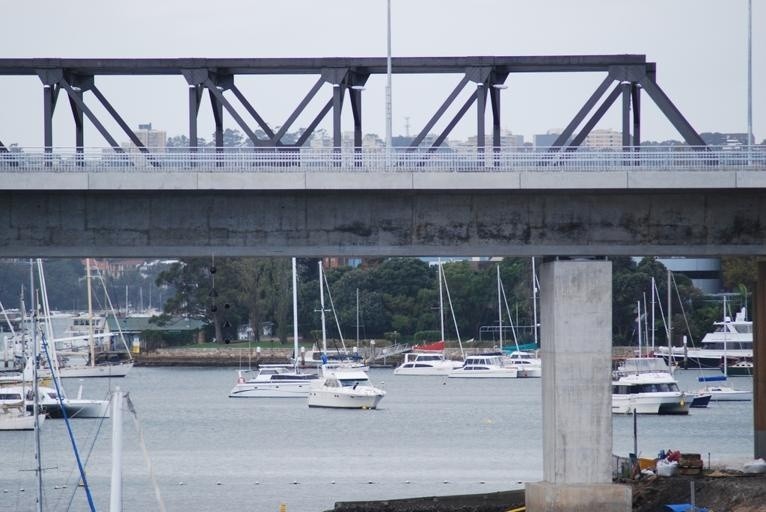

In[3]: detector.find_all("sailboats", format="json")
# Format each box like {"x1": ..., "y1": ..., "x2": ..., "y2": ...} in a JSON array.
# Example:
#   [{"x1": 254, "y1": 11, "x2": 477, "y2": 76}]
[
  {"x1": 445, "y1": 264, "x2": 520, "y2": 379},
  {"x1": 506, "y1": 256, "x2": 543, "y2": 379},
  {"x1": 392, "y1": 257, "x2": 473, "y2": 377},
  {"x1": 609, "y1": 269, "x2": 754, "y2": 415},
  {"x1": 230, "y1": 256, "x2": 322, "y2": 399},
  {"x1": 0, "y1": 257, "x2": 137, "y2": 432},
  {"x1": 306, "y1": 259, "x2": 386, "y2": 409}
]
